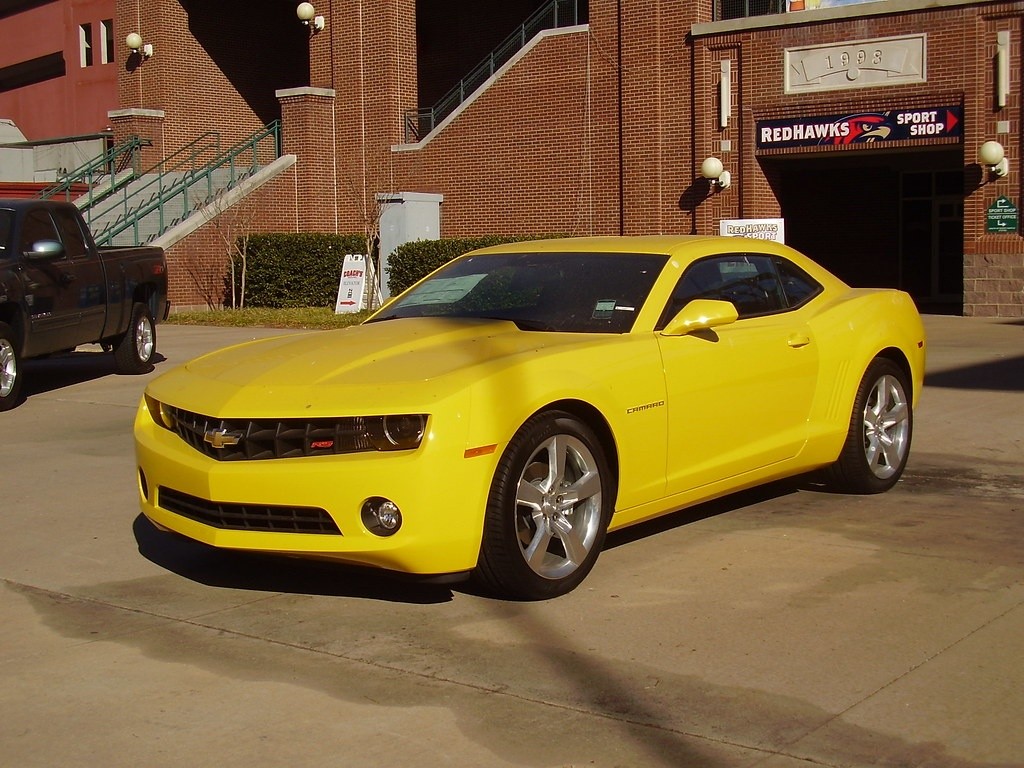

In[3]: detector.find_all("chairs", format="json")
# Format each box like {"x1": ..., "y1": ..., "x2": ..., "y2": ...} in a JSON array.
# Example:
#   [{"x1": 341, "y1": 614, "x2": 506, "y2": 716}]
[{"x1": 637, "y1": 260, "x2": 725, "y2": 319}]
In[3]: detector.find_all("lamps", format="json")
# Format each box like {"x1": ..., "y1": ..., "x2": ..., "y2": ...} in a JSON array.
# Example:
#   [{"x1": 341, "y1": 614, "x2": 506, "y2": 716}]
[
  {"x1": 979, "y1": 141, "x2": 1007, "y2": 177},
  {"x1": 126, "y1": 33, "x2": 153, "y2": 57},
  {"x1": 297, "y1": 2, "x2": 324, "y2": 30},
  {"x1": 701, "y1": 157, "x2": 731, "y2": 189}
]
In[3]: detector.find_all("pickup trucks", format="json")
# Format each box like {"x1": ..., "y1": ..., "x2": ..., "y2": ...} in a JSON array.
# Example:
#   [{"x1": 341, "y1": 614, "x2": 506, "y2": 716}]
[{"x1": 0, "y1": 195, "x2": 170, "y2": 410}]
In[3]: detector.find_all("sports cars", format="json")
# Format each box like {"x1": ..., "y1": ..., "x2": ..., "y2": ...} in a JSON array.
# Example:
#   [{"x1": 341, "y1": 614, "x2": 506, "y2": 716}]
[{"x1": 130, "y1": 236, "x2": 928, "y2": 606}]
